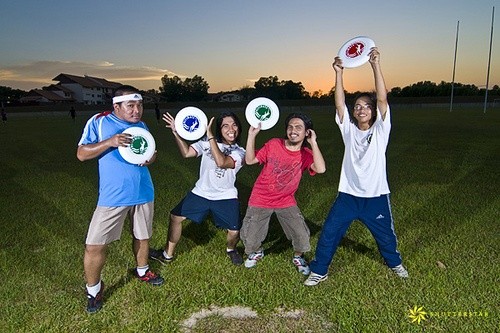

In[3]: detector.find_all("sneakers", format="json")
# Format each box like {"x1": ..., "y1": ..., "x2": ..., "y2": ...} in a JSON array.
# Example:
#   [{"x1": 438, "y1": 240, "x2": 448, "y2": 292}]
[
  {"x1": 149, "y1": 248, "x2": 173, "y2": 266},
  {"x1": 134, "y1": 268, "x2": 164, "y2": 285},
  {"x1": 244, "y1": 250, "x2": 264, "y2": 267},
  {"x1": 227, "y1": 249, "x2": 243, "y2": 265},
  {"x1": 303, "y1": 270, "x2": 328, "y2": 285},
  {"x1": 392, "y1": 263, "x2": 408, "y2": 277},
  {"x1": 292, "y1": 255, "x2": 311, "y2": 275},
  {"x1": 87, "y1": 280, "x2": 105, "y2": 312}
]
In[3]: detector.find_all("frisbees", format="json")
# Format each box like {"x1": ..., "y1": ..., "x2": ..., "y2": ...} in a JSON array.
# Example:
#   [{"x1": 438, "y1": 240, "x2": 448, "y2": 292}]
[
  {"x1": 175, "y1": 106, "x2": 208, "y2": 141},
  {"x1": 245, "y1": 97, "x2": 280, "y2": 131},
  {"x1": 118, "y1": 127, "x2": 156, "y2": 165},
  {"x1": 337, "y1": 36, "x2": 375, "y2": 69}
]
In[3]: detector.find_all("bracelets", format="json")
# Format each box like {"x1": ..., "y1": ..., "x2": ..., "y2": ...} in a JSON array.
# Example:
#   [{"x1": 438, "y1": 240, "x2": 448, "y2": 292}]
[{"x1": 207, "y1": 136, "x2": 216, "y2": 142}]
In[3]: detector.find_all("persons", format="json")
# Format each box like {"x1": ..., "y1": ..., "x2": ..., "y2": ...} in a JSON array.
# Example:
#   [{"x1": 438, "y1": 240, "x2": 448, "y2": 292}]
[
  {"x1": 68, "y1": 106, "x2": 76, "y2": 121},
  {"x1": 303, "y1": 47, "x2": 408, "y2": 286},
  {"x1": 239, "y1": 114, "x2": 327, "y2": 275},
  {"x1": 1, "y1": 107, "x2": 8, "y2": 128},
  {"x1": 155, "y1": 103, "x2": 161, "y2": 122},
  {"x1": 148, "y1": 111, "x2": 246, "y2": 266},
  {"x1": 76, "y1": 85, "x2": 164, "y2": 313}
]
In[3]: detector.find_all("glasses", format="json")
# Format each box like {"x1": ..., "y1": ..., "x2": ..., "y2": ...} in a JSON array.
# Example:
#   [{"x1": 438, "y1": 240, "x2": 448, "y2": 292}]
[{"x1": 354, "y1": 105, "x2": 371, "y2": 110}]
